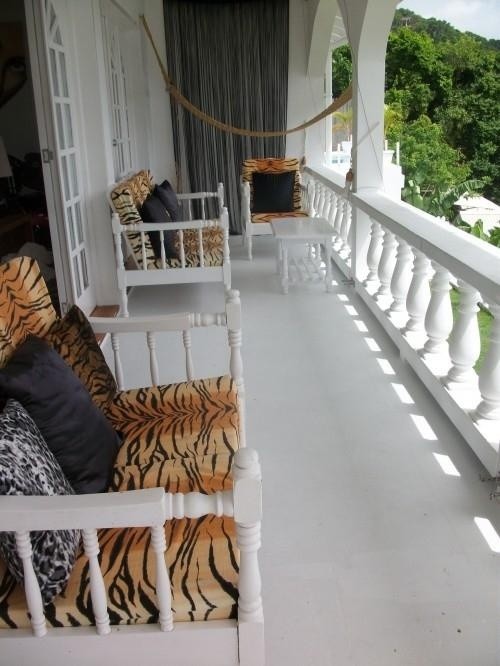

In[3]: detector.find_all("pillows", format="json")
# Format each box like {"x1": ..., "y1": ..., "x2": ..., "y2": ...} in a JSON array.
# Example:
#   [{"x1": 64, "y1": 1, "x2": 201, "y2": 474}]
[
  {"x1": 153, "y1": 179, "x2": 181, "y2": 234},
  {"x1": 44, "y1": 304, "x2": 117, "y2": 414},
  {"x1": 1, "y1": 399, "x2": 85, "y2": 608},
  {"x1": 250, "y1": 169, "x2": 297, "y2": 214},
  {"x1": 142, "y1": 193, "x2": 177, "y2": 262},
  {"x1": 2, "y1": 333, "x2": 123, "y2": 498}
]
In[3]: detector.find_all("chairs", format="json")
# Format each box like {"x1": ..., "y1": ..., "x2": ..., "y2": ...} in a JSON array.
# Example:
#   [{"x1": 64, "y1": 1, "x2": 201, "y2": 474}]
[{"x1": 238, "y1": 156, "x2": 316, "y2": 260}]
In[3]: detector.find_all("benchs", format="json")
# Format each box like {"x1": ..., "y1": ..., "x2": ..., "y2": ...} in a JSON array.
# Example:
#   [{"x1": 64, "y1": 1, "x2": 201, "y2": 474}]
[
  {"x1": 1, "y1": 256, "x2": 267, "y2": 664},
  {"x1": 106, "y1": 168, "x2": 233, "y2": 318}
]
